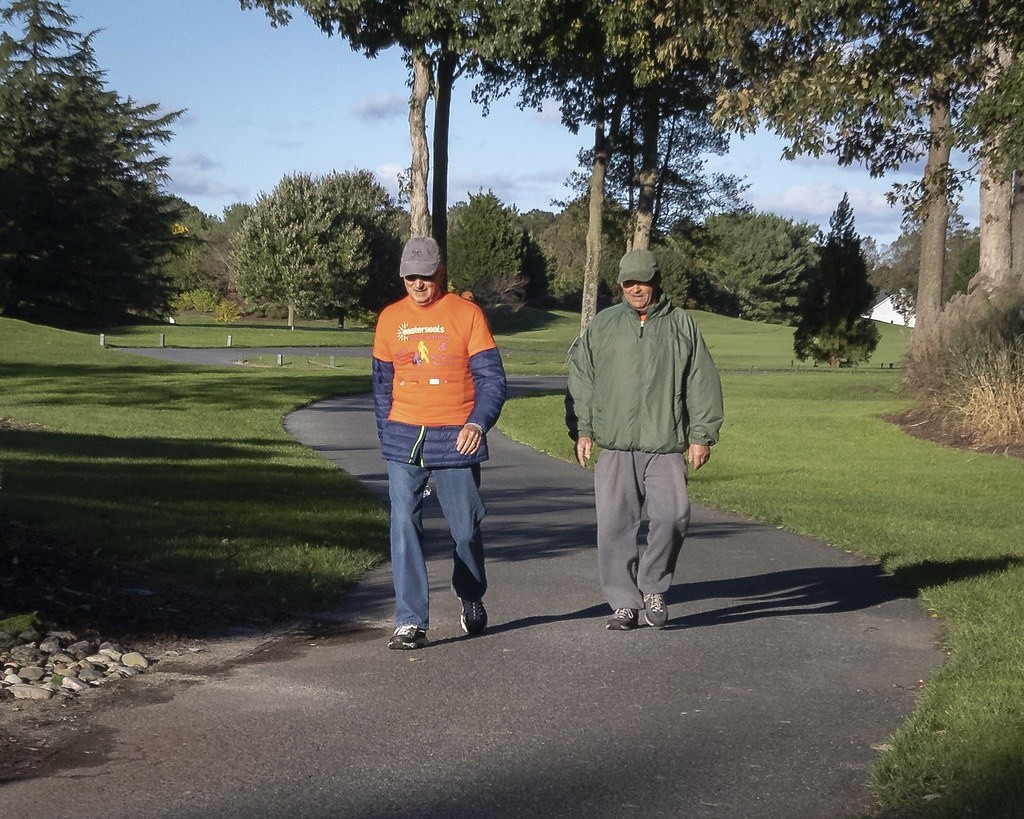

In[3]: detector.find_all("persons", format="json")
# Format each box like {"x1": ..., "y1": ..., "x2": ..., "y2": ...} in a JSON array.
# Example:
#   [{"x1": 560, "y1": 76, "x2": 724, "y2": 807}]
[
  {"x1": 370, "y1": 236, "x2": 507, "y2": 650},
  {"x1": 563, "y1": 250, "x2": 725, "y2": 631}
]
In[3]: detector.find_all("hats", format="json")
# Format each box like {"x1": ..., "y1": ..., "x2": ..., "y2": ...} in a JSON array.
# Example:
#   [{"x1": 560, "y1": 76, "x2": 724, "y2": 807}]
[
  {"x1": 618, "y1": 250, "x2": 659, "y2": 283},
  {"x1": 399, "y1": 236, "x2": 441, "y2": 278}
]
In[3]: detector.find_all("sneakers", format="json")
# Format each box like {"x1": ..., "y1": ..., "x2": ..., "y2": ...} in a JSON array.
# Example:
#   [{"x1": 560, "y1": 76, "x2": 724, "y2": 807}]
[
  {"x1": 388, "y1": 624, "x2": 430, "y2": 649},
  {"x1": 642, "y1": 592, "x2": 669, "y2": 628},
  {"x1": 450, "y1": 584, "x2": 489, "y2": 635},
  {"x1": 606, "y1": 607, "x2": 639, "y2": 631}
]
